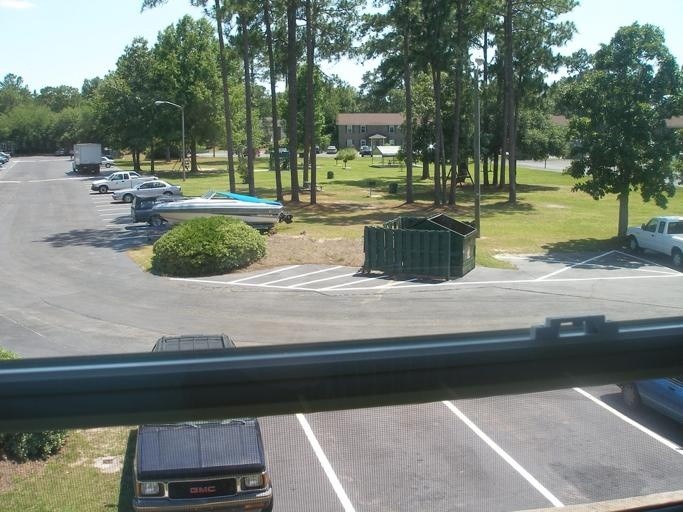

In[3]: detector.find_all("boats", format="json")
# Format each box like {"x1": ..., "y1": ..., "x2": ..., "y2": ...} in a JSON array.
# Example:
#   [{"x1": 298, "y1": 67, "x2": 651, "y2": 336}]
[{"x1": 152, "y1": 189, "x2": 292, "y2": 235}]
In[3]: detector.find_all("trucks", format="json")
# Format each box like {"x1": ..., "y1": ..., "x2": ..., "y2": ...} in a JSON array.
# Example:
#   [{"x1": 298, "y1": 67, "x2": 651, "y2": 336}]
[{"x1": 72, "y1": 143, "x2": 101, "y2": 174}]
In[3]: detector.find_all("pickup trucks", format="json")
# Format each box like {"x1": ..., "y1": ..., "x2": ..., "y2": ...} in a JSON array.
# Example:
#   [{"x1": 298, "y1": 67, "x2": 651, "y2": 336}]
[
  {"x1": 625, "y1": 216, "x2": 682, "y2": 267},
  {"x1": 90, "y1": 170, "x2": 158, "y2": 193}
]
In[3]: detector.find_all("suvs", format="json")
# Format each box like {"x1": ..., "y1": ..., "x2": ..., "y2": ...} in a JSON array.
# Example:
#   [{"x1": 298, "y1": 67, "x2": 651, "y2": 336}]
[
  {"x1": 130, "y1": 194, "x2": 188, "y2": 226},
  {"x1": 131, "y1": 332, "x2": 273, "y2": 512},
  {"x1": 361, "y1": 147, "x2": 373, "y2": 157}
]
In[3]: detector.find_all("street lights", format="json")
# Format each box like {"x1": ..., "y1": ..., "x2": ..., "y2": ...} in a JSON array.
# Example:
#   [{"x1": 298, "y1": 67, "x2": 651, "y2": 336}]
[
  {"x1": 154, "y1": 100, "x2": 187, "y2": 181},
  {"x1": 471, "y1": 56, "x2": 485, "y2": 239}
]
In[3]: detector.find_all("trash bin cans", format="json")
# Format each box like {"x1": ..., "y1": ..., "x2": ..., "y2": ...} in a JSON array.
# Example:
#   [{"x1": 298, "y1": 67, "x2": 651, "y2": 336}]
[{"x1": 405, "y1": 212, "x2": 477, "y2": 277}]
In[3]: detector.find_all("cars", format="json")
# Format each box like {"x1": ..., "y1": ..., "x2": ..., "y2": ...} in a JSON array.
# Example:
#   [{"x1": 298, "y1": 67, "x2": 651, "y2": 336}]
[
  {"x1": 233, "y1": 145, "x2": 337, "y2": 158},
  {"x1": 359, "y1": 145, "x2": 369, "y2": 153},
  {"x1": 0, "y1": 151, "x2": 11, "y2": 162},
  {"x1": 0, "y1": 155, "x2": 7, "y2": 166},
  {"x1": 54, "y1": 147, "x2": 73, "y2": 155},
  {"x1": 100, "y1": 156, "x2": 115, "y2": 168},
  {"x1": 104, "y1": 171, "x2": 142, "y2": 179},
  {"x1": 112, "y1": 179, "x2": 181, "y2": 203},
  {"x1": 0, "y1": 163, "x2": 3, "y2": 168},
  {"x1": 617, "y1": 376, "x2": 683, "y2": 425}
]
